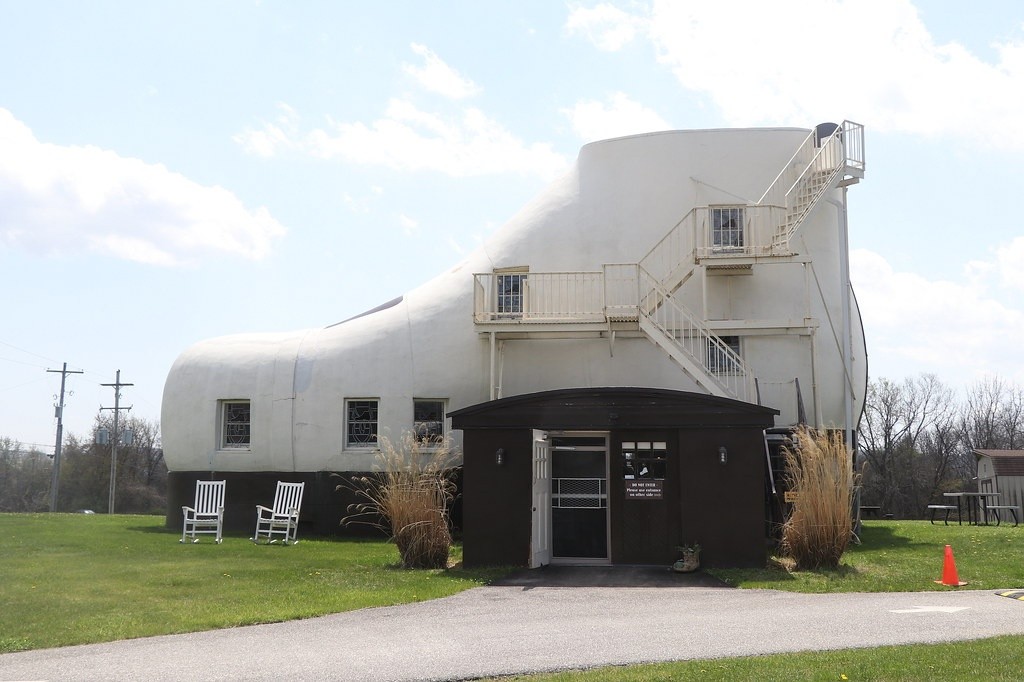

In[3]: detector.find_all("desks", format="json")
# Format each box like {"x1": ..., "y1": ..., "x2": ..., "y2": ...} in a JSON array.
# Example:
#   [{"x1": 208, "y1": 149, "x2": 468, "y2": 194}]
[
  {"x1": 860, "y1": 505, "x2": 882, "y2": 520},
  {"x1": 943, "y1": 492, "x2": 1001, "y2": 526}
]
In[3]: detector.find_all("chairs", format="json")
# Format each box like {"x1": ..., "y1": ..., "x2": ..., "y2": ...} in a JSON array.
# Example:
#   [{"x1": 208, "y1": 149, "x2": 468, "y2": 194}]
[
  {"x1": 249, "y1": 480, "x2": 305, "y2": 546},
  {"x1": 179, "y1": 480, "x2": 226, "y2": 544}
]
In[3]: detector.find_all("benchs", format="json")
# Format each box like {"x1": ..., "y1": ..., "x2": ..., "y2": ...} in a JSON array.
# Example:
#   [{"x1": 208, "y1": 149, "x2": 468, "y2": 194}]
[
  {"x1": 928, "y1": 505, "x2": 957, "y2": 526},
  {"x1": 986, "y1": 505, "x2": 1020, "y2": 527},
  {"x1": 882, "y1": 514, "x2": 894, "y2": 519}
]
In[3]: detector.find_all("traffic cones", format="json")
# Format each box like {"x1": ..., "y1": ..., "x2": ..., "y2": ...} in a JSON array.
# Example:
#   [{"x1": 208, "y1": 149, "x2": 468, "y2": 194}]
[{"x1": 934, "y1": 545, "x2": 969, "y2": 586}]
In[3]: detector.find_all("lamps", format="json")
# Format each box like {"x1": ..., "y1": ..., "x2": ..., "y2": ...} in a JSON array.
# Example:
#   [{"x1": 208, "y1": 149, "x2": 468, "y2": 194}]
[
  {"x1": 718, "y1": 446, "x2": 728, "y2": 466},
  {"x1": 495, "y1": 448, "x2": 504, "y2": 466}
]
